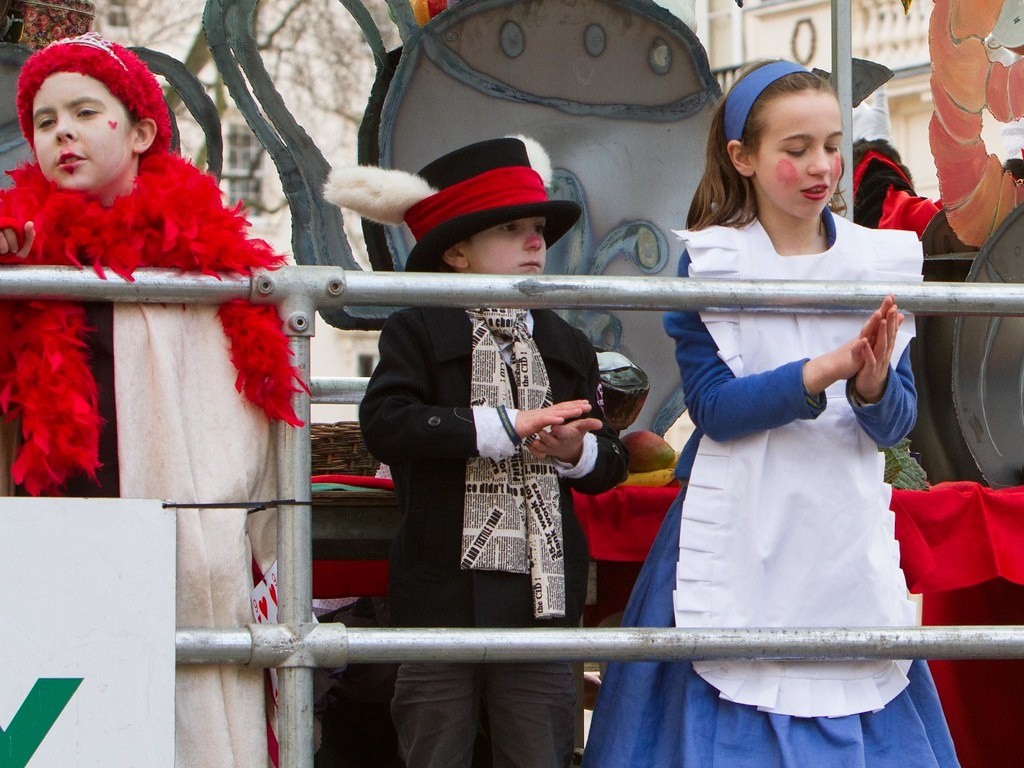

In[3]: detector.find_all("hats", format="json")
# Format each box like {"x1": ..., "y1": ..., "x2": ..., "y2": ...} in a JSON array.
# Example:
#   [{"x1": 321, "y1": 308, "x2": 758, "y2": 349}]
[
  {"x1": 16, "y1": 31, "x2": 172, "y2": 160},
  {"x1": 321, "y1": 133, "x2": 582, "y2": 272}
]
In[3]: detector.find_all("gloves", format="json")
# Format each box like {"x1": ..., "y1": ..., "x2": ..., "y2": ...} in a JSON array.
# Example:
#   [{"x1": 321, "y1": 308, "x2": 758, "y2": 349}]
[{"x1": 851, "y1": 83, "x2": 894, "y2": 148}]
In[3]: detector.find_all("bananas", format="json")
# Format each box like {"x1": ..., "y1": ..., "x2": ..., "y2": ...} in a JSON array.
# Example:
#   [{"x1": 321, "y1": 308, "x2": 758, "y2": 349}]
[{"x1": 617, "y1": 452, "x2": 681, "y2": 487}]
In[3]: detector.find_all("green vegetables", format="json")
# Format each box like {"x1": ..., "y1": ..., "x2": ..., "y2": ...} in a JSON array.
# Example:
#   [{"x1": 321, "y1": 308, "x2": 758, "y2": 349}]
[{"x1": 877, "y1": 438, "x2": 928, "y2": 491}]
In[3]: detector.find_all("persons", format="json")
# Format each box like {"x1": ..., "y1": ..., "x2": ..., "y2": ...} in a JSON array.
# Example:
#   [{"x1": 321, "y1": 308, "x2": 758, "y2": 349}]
[
  {"x1": 576, "y1": 59, "x2": 961, "y2": 768},
  {"x1": 353, "y1": 136, "x2": 628, "y2": 768},
  {"x1": 0, "y1": 31, "x2": 315, "y2": 768},
  {"x1": 855, "y1": 82, "x2": 1023, "y2": 240}
]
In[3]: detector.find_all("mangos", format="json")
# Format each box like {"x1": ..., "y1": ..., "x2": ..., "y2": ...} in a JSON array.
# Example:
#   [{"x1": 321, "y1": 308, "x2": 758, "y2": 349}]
[{"x1": 621, "y1": 430, "x2": 675, "y2": 473}]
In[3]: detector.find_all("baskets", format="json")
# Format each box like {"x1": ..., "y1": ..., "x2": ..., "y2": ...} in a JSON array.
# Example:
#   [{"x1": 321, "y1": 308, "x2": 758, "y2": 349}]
[{"x1": 307, "y1": 420, "x2": 379, "y2": 478}]
